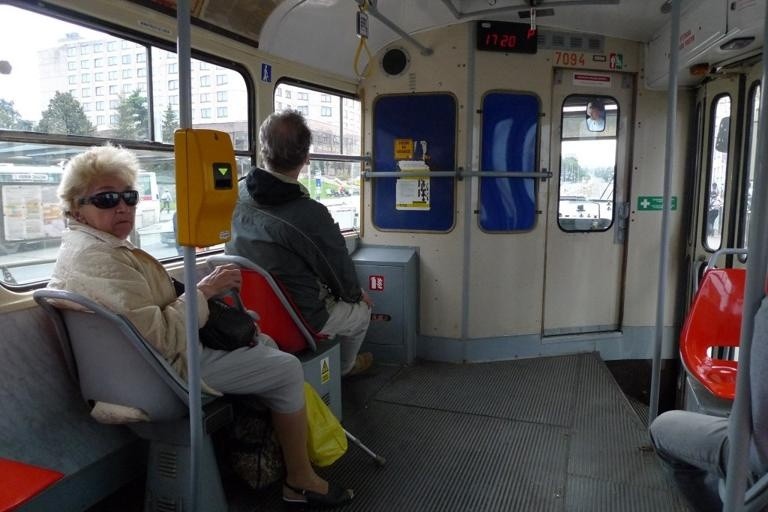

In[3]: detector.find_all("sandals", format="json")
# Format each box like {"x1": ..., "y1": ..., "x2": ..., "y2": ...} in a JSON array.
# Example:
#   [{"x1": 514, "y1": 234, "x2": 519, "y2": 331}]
[{"x1": 281, "y1": 481, "x2": 355, "y2": 505}]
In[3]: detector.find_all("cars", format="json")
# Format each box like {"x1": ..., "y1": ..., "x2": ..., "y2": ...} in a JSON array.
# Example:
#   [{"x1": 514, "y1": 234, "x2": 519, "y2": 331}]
[{"x1": 159, "y1": 221, "x2": 175, "y2": 245}]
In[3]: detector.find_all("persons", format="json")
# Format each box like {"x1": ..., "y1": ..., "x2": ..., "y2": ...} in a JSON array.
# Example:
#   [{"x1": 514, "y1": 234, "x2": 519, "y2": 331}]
[
  {"x1": 587, "y1": 104, "x2": 603, "y2": 130},
  {"x1": 337, "y1": 183, "x2": 344, "y2": 194},
  {"x1": 160, "y1": 189, "x2": 173, "y2": 213},
  {"x1": 644, "y1": 294, "x2": 767, "y2": 501},
  {"x1": 710, "y1": 183, "x2": 719, "y2": 225},
  {"x1": 224, "y1": 106, "x2": 374, "y2": 377},
  {"x1": 43, "y1": 140, "x2": 355, "y2": 506},
  {"x1": 313, "y1": 169, "x2": 321, "y2": 190}
]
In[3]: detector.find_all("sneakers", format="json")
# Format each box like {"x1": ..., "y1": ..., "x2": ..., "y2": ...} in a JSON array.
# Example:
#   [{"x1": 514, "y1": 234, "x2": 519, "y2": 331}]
[{"x1": 352, "y1": 350, "x2": 374, "y2": 376}]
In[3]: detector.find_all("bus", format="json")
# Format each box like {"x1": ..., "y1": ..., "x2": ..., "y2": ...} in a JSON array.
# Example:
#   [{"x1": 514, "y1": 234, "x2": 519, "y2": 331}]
[{"x1": 0, "y1": 162, "x2": 161, "y2": 286}]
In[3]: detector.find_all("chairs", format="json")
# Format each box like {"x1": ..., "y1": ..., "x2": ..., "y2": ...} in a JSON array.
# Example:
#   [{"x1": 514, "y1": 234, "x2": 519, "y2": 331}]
[
  {"x1": 206, "y1": 253, "x2": 345, "y2": 427},
  {"x1": 676, "y1": 247, "x2": 767, "y2": 402},
  {"x1": 33, "y1": 287, "x2": 253, "y2": 510}
]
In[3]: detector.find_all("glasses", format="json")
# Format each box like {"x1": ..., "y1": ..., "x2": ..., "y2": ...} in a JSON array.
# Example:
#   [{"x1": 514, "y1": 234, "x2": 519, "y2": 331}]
[{"x1": 77, "y1": 190, "x2": 139, "y2": 209}]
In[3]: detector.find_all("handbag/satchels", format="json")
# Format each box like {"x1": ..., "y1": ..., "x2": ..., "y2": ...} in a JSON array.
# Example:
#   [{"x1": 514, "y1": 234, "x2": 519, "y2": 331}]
[
  {"x1": 171, "y1": 277, "x2": 256, "y2": 351},
  {"x1": 302, "y1": 382, "x2": 348, "y2": 467},
  {"x1": 234, "y1": 411, "x2": 287, "y2": 493}
]
